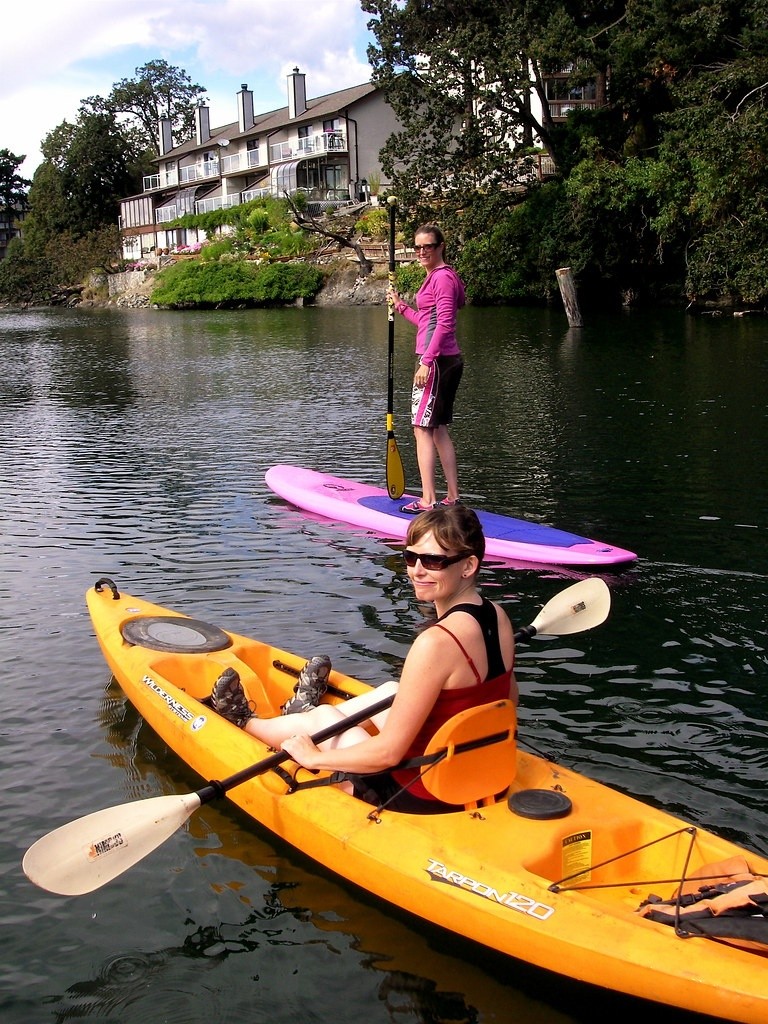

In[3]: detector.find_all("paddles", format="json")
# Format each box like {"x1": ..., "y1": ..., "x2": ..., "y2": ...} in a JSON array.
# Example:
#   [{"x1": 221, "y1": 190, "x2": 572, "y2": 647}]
[
  {"x1": 21, "y1": 577, "x2": 612, "y2": 897},
  {"x1": 385, "y1": 196, "x2": 405, "y2": 500}
]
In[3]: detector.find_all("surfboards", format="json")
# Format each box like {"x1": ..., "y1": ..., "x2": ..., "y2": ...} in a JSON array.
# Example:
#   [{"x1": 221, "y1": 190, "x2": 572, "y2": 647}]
[{"x1": 265, "y1": 465, "x2": 637, "y2": 564}]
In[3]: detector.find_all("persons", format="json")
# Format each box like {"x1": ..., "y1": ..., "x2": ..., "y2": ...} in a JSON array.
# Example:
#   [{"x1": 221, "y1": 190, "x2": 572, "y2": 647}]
[
  {"x1": 210, "y1": 506, "x2": 514, "y2": 817},
  {"x1": 385, "y1": 225, "x2": 466, "y2": 514}
]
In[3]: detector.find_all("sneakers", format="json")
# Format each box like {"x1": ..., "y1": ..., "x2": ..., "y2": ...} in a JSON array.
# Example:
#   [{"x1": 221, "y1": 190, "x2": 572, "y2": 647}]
[
  {"x1": 210, "y1": 668, "x2": 257, "y2": 729},
  {"x1": 398, "y1": 496, "x2": 460, "y2": 514},
  {"x1": 280, "y1": 654, "x2": 334, "y2": 716}
]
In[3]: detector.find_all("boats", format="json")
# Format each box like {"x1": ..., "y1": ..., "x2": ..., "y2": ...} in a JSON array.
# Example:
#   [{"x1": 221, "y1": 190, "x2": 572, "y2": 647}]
[{"x1": 86, "y1": 578, "x2": 768, "y2": 1024}]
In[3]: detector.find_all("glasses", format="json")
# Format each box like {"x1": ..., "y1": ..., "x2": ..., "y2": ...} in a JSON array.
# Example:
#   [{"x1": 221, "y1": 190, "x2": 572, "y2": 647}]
[
  {"x1": 413, "y1": 243, "x2": 441, "y2": 253},
  {"x1": 403, "y1": 549, "x2": 472, "y2": 572}
]
